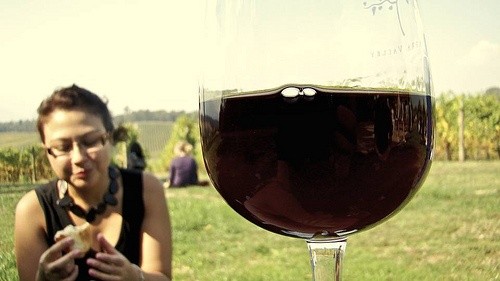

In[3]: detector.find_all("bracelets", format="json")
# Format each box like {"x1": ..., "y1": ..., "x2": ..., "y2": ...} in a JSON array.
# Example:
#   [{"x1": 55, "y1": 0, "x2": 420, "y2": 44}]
[{"x1": 133, "y1": 263, "x2": 145, "y2": 280}]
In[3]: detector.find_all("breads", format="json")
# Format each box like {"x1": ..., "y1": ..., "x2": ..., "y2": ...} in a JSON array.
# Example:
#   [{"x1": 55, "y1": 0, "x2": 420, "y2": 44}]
[{"x1": 53, "y1": 222, "x2": 92, "y2": 258}]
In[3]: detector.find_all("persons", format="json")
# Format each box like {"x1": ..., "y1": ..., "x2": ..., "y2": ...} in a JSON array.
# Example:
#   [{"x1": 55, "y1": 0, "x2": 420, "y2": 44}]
[
  {"x1": 163, "y1": 141, "x2": 199, "y2": 188},
  {"x1": 14, "y1": 83, "x2": 174, "y2": 280}
]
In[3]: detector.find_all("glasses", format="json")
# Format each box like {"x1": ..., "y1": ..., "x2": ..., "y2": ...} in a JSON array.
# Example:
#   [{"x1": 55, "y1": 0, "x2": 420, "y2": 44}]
[{"x1": 47, "y1": 130, "x2": 111, "y2": 158}]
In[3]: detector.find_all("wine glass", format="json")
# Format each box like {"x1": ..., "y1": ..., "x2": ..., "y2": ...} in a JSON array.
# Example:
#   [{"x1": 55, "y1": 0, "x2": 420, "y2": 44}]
[{"x1": 198, "y1": 1, "x2": 435, "y2": 281}]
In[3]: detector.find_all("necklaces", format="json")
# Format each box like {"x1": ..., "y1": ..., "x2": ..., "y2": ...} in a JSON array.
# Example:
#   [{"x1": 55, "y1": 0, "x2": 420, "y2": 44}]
[{"x1": 55, "y1": 167, "x2": 121, "y2": 221}]
